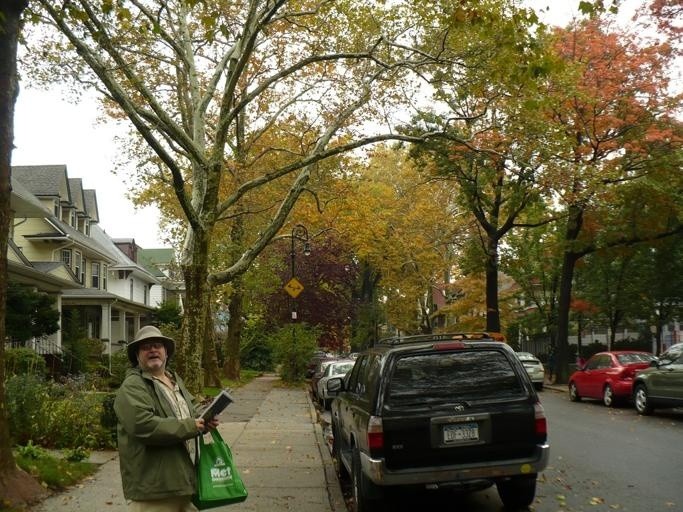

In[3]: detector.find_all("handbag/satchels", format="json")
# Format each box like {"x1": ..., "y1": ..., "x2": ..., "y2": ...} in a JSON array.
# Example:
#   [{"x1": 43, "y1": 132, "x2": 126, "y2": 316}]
[{"x1": 192, "y1": 442, "x2": 247, "y2": 510}]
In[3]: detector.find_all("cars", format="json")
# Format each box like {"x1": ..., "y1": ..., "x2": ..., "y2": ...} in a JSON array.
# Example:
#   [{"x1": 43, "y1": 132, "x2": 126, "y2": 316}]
[
  {"x1": 307, "y1": 348, "x2": 361, "y2": 410},
  {"x1": 565, "y1": 348, "x2": 659, "y2": 408},
  {"x1": 631, "y1": 342, "x2": 683, "y2": 416},
  {"x1": 511, "y1": 349, "x2": 546, "y2": 392}
]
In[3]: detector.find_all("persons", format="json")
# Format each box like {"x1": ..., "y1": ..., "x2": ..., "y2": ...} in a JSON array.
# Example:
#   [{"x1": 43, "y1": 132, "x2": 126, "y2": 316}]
[{"x1": 111, "y1": 324, "x2": 220, "y2": 511}]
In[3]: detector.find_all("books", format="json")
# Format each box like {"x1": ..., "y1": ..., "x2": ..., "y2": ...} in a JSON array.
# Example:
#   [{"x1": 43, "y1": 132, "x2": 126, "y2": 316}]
[{"x1": 198, "y1": 390, "x2": 234, "y2": 436}]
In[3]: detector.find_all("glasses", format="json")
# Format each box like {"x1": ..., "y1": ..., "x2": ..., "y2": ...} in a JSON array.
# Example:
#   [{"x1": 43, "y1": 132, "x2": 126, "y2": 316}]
[{"x1": 141, "y1": 343, "x2": 165, "y2": 352}]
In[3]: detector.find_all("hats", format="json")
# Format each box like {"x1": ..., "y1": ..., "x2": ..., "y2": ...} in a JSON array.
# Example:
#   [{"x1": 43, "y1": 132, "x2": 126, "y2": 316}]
[{"x1": 128, "y1": 326, "x2": 174, "y2": 366}]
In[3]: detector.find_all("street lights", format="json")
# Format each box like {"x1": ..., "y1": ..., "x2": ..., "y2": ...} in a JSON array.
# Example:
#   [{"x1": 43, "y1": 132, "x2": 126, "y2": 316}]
[{"x1": 290, "y1": 222, "x2": 311, "y2": 383}]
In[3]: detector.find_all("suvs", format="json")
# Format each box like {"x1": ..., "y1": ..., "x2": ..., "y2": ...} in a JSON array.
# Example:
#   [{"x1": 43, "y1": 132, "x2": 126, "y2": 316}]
[{"x1": 328, "y1": 330, "x2": 551, "y2": 512}]
